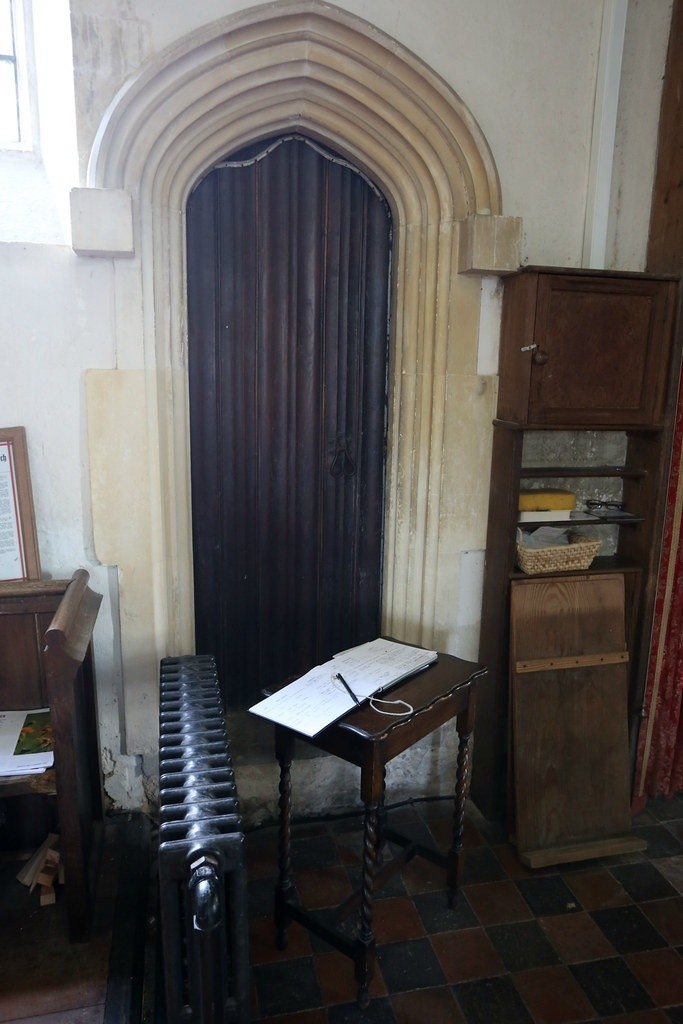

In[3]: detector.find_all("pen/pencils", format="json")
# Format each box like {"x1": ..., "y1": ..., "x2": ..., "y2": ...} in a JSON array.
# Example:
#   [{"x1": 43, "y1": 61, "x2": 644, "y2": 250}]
[{"x1": 336, "y1": 673, "x2": 361, "y2": 707}]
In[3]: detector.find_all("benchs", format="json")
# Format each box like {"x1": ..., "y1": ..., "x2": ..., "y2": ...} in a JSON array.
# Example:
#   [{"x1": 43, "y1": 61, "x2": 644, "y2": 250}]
[{"x1": 0, "y1": 570, "x2": 107, "y2": 947}]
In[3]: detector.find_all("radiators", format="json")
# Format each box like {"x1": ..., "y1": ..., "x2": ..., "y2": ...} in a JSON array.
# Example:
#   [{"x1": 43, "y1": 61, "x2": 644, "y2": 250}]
[{"x1": 156, "y1": 653, "x2": 250, "y2": 1024}]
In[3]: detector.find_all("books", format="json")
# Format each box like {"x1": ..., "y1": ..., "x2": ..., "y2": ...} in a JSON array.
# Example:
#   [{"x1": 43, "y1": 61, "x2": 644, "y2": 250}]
[
  {"x1": 247, "y1": 636, "x2": 438, "y2": 743},
  {"x1": 0, "y1": 708, "x2": 55, "y2": 777}
]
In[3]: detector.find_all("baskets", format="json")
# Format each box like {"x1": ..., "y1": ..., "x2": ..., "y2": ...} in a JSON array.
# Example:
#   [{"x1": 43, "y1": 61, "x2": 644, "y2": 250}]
[{"x1": 515, "y1": 526, "x2": 602, "y2": 575}]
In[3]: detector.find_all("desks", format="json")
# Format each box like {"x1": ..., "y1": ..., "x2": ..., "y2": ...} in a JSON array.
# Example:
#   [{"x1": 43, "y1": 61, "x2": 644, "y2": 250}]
[{"x1": 260, "y1": 637, "x2": 489, "y2": 1011}]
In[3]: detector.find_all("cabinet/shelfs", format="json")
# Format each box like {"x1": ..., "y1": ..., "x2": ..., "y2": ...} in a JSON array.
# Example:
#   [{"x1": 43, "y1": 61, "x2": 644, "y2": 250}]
[{"x1": 467, "y1": 266, "x2": 683, "y2": 820}]
